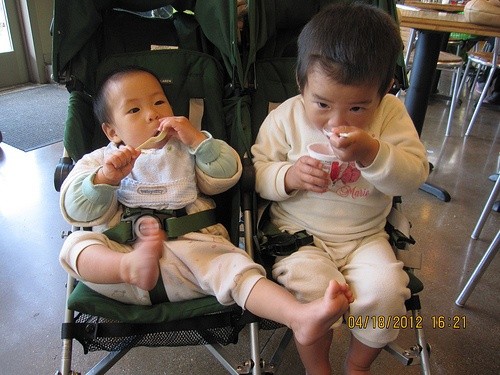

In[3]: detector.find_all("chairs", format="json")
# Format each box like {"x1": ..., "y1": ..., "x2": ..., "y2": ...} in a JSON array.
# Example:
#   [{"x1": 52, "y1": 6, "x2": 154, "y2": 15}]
[{"x1": 399, "y1": 0, "x2": 500, "y2": 307}]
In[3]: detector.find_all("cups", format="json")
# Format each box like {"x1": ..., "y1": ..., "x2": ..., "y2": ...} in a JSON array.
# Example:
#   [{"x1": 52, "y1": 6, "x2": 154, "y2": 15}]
[{"x1": 308, "y1": 142, "x2": 344, "y2": 189}]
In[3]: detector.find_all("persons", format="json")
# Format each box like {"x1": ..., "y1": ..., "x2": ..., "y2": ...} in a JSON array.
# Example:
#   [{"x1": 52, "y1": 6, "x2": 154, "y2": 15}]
[
  {"x1": 59, "y1": 66, "x2": 355, "y2": 345},
  {"x1": 249, "y1": 2, "x2": 429, "y2": 375}
]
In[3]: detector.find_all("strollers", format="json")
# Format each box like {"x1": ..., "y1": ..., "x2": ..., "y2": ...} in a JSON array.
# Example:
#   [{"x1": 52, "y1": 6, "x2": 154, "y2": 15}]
[{"x1": 48, "y1": 0, "x2": 433, "y2": 375}]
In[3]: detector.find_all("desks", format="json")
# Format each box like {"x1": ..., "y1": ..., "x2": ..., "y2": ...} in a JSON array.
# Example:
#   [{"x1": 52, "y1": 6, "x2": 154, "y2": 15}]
[{"x1": 397, "y1": 6, "x2": 500, "y2": 202}]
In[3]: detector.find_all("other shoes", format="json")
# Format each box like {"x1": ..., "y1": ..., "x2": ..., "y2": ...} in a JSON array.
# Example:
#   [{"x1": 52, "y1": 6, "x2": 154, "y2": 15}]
[{"x1": 480, "y1": 92, "x2": 500, "y2": 105}]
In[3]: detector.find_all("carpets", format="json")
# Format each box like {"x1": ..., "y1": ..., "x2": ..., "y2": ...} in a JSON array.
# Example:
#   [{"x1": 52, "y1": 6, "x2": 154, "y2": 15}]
[{"x1": 0, "y1": 84, "x2": 70, "y2": 152}]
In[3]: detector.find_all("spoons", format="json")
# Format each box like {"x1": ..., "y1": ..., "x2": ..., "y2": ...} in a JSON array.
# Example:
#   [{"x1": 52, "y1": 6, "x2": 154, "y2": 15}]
[{"x1": 135, "y1": 127, "x2": 170, "y2": 150}]
